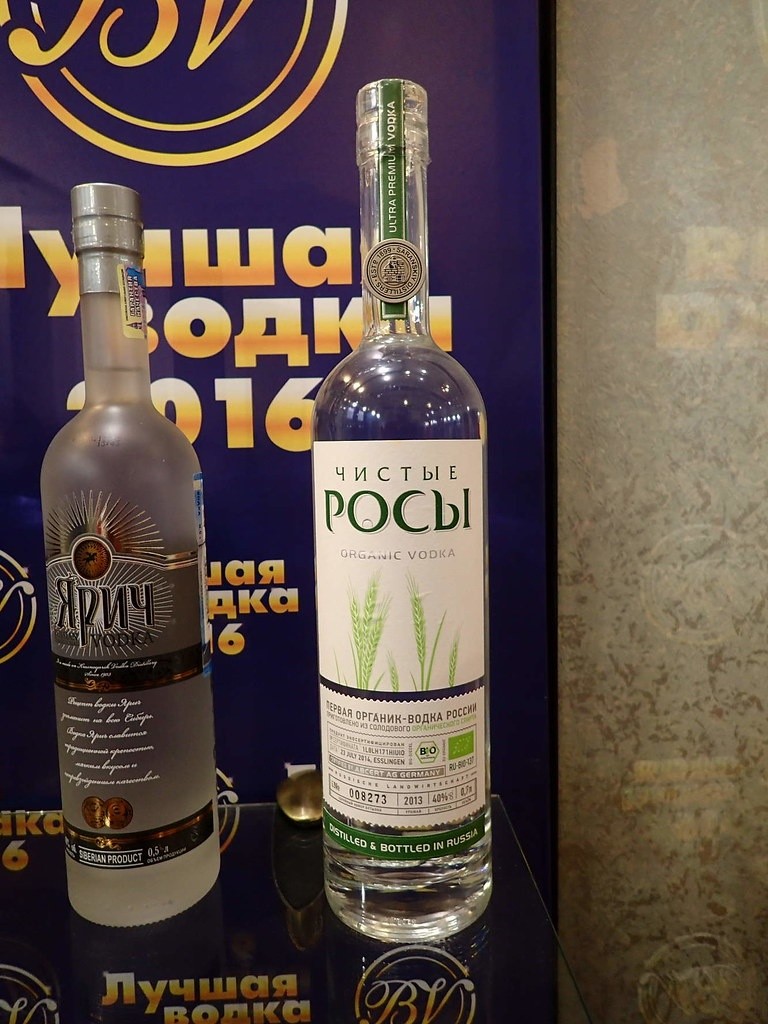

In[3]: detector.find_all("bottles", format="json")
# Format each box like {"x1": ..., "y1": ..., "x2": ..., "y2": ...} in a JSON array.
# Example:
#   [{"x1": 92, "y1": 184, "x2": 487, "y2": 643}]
[
  {"x1": 312, "y1": 77, "x2": 494, "y2": 944},
  {"x1": 40, "y1": 183, "x2": 220, "y2": 927}
]
